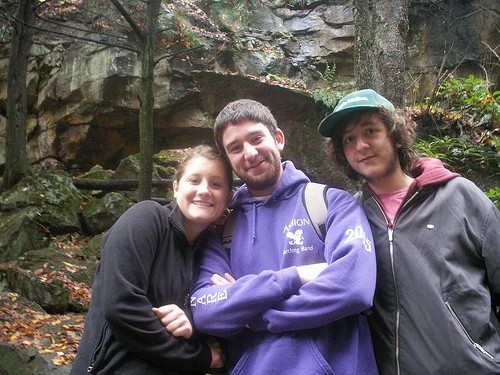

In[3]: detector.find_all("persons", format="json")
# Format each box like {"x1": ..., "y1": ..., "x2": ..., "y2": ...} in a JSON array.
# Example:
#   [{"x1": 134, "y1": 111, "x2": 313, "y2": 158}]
[
  {"x1": 317, "y1": 89, "x2": 500, "y2": 375},
  {"x1": 191, "y1": 99, "x2": 379, "y2": 375},
  {"x1": 68, "y1": 145, "x2": 234, "y2": 375}
]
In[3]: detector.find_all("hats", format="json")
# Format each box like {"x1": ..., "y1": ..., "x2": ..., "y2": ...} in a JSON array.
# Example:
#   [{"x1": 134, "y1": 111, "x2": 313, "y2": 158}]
[{"x1": 317, "y1": 88, "x2": 399, "y2": 138}]
